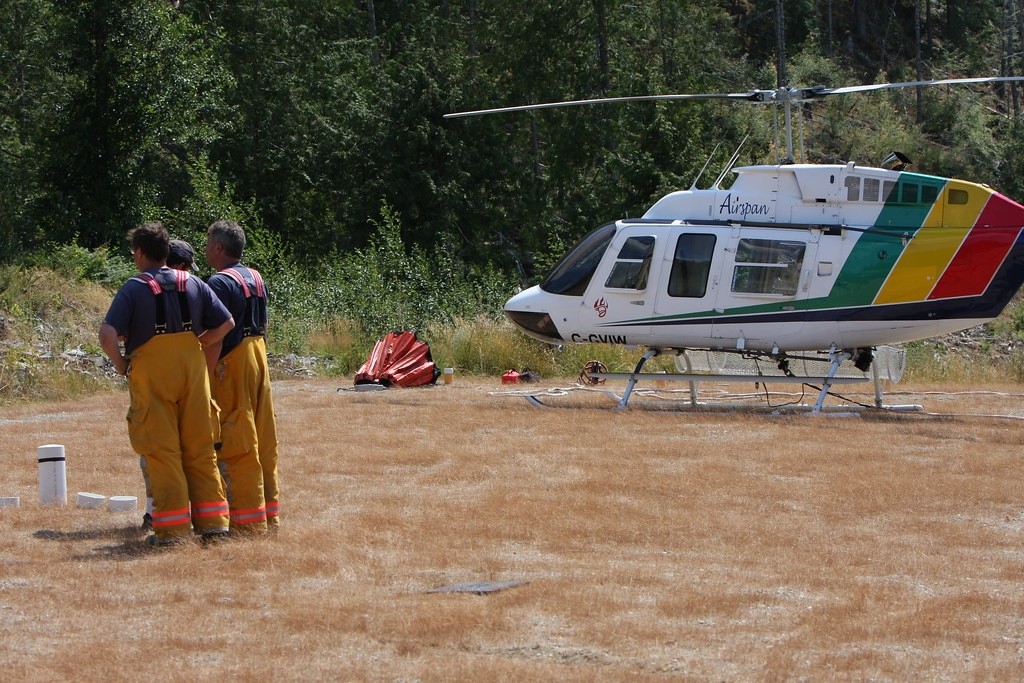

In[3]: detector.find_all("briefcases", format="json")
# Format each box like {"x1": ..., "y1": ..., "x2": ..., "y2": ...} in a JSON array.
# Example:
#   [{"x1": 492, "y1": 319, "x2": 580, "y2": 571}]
[{"x1": 502, "y1": 370, "x2": 519, "y2": 385}]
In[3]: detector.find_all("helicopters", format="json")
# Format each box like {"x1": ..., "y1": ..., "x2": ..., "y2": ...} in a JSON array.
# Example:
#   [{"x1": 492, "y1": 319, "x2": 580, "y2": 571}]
[{"x1": 443, "y1": 75, "x2": 1024, "y2": 419}]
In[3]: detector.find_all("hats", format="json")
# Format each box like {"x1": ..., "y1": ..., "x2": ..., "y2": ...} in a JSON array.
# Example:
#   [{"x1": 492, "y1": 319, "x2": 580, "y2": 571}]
[{"x1": 167, "y1": 239, "x2": 200, "y2": 272}]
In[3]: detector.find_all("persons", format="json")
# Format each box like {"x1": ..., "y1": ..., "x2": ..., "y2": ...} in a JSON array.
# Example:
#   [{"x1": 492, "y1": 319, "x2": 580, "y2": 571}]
[{"x1": 99, "y1": 221, "x2": 283, "y2": 548}]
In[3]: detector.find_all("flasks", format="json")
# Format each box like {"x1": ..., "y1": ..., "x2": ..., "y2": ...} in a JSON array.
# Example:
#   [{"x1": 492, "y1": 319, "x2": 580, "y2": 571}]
[{"x1": 37, "y1": 444, "x2": 67, "y2": 505}]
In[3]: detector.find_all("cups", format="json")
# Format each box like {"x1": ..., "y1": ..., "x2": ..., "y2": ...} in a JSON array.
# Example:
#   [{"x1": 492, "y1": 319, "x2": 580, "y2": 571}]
[
  {"x1": 0, "y1": 496, "x2": 20, "y2": 510},
  {"x1": 77, "y1": 492, "x2": 106, "y2": 509},
  {"x1": 445, "y1": 367, "x2": 453, "y2": 385},
  {"x1": 110, "y1": 496, "x2": 137, "y2": 512}
]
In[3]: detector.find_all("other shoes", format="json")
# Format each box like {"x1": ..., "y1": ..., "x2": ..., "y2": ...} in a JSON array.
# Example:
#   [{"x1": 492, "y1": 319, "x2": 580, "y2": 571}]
[
  {"x1": 199, "y1": 532, "x2": 228, "y2": 547},
  {"x1": 145, "y1": 534, "x2": 184, "y2": 551},
  {"x1": 141, "y1": 513, "x2": 153, "y2": 530}
]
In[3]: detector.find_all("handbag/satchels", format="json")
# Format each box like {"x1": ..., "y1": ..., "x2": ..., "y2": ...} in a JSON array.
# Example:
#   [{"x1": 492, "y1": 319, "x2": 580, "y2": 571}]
[{"x1": 520, "y1": 367, "x2": 540, "y2": 383}]
